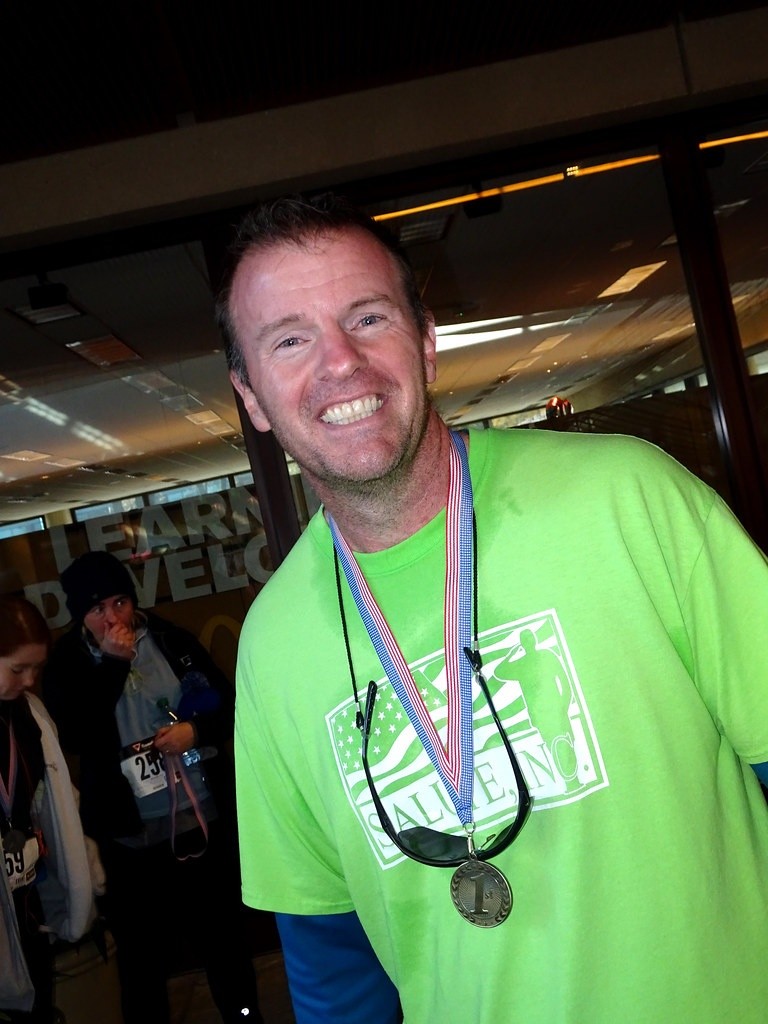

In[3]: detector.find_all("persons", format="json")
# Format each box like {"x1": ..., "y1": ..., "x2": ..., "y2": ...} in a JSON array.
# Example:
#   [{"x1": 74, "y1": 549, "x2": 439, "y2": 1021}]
[
  {"x1": 0, "y1": 591, "x2": 107, "y2": 1024},
  {"x1": 220, "y1": 187, "x2": 767, "y2": 1024},
  {"x1": 36, "y1": 549, "x2": 249, "y2": 1024}
]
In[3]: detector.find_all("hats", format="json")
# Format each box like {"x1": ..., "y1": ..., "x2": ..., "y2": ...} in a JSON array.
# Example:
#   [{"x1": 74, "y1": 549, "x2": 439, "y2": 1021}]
[{"x1": 58, "y1": 551, "x2": 140, "y2": 624}]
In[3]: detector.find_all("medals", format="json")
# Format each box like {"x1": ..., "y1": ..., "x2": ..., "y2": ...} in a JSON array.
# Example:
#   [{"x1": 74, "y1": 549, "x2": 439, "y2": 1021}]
[{"x1": 450, "y1": 859, "x2": 514, "y2": 928}]
